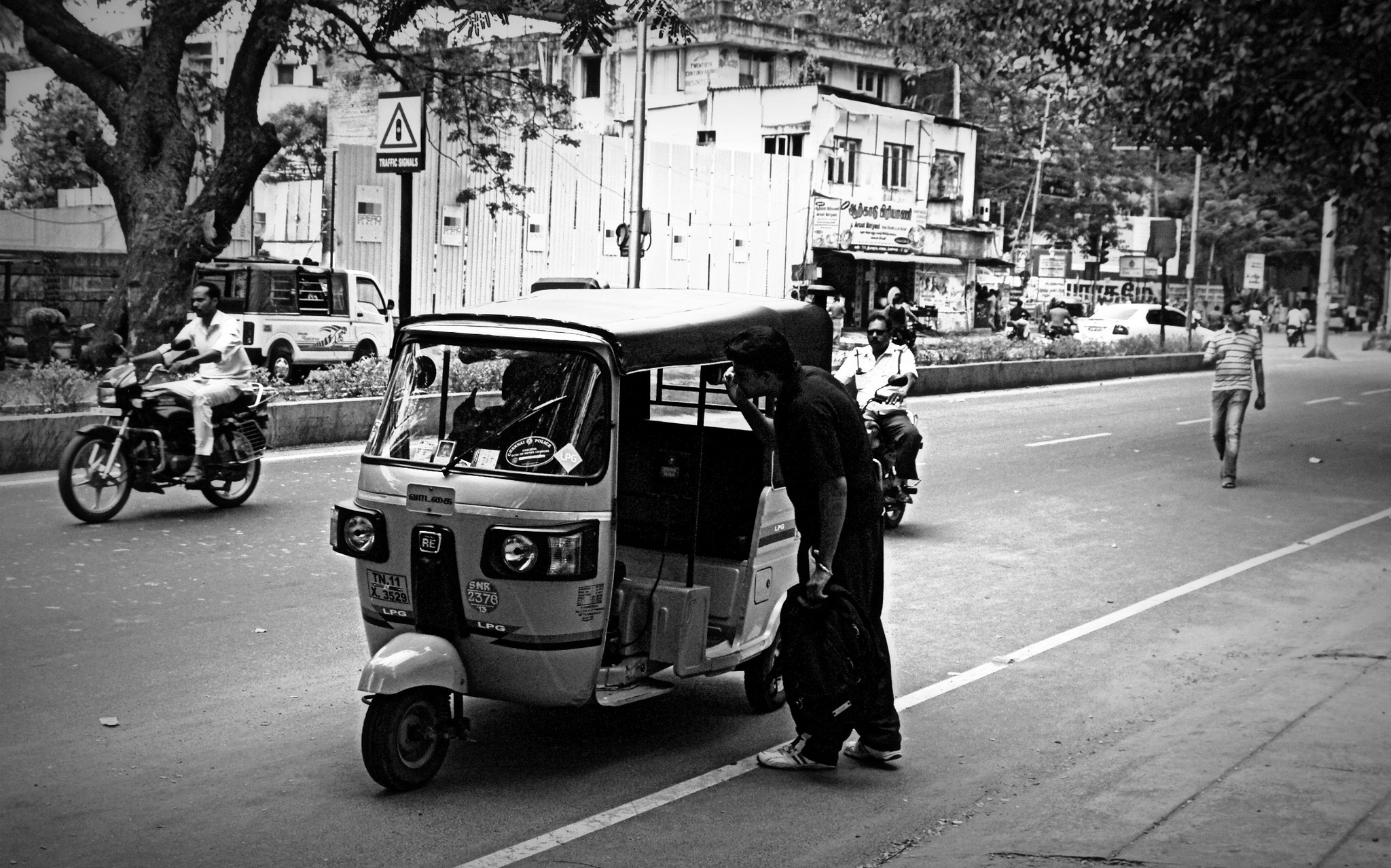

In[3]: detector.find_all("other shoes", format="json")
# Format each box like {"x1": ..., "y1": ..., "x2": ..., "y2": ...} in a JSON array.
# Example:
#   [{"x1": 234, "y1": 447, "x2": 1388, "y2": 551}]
[{"x1": 1222, "y1": 478, "x2": 1234, "y2": 488}]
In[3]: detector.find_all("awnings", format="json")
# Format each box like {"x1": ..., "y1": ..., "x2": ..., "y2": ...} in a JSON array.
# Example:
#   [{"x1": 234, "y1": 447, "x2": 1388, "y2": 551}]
[{"x1": 834, "y1": 250, "x2": 962, "y2": 276}]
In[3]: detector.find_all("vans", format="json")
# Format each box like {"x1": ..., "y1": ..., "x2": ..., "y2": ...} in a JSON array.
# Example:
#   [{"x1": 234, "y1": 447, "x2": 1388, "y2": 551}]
[{"x1": 192, "y1": 258, "x2": 397, "y2": 386}]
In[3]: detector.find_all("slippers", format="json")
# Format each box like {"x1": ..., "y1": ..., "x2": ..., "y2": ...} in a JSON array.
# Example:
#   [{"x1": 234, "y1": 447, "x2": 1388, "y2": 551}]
[{"x1": 181, "y1": 471, "x2": 207, "y2": 483}]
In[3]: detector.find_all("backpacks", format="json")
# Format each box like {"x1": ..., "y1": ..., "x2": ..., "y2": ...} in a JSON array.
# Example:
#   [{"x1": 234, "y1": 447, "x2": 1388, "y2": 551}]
[{"x1": 776, "y1": 580, "x2": 871, "y2": 733}]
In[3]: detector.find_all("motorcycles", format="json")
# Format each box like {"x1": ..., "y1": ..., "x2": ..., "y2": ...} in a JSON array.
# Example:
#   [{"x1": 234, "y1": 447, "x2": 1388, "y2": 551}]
[
  {"x1": 1005, "y1": 315, "x2": 1030, "y2": 342},
  {"x1": 58, "y1": 332, "x2": 279, "y2": 523},
  {"x1": 328, "y1": 284, "x2": 834, "y2": 792},
  {"x1": 891, "y1": 324, "x2": 923, "y2": 354},
  {"x1": 856, "y1": 373, "x2": 923, "y2": 528},
  {"x1": 1039, "y1": 316, "x2": 1079, "y2": 346}
]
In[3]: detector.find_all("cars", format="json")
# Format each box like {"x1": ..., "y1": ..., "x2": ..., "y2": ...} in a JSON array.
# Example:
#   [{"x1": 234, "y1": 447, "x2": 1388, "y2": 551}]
[{"x1": 1071, "y1": 303, "x2": 1217, "y2": 352}]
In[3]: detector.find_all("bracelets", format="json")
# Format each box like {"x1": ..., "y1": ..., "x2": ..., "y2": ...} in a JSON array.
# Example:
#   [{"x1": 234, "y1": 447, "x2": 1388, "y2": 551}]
[
  {"x1": 819, "y1": 564, "x2": 833, "y2": 576},
  {"x1": 1259, "y1": 391, "x2": 1265, "y2": 395}
]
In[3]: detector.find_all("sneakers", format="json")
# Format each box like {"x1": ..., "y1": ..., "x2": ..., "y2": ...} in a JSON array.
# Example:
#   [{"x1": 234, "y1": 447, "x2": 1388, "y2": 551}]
[
  {"x1": 844, "y1": 740, "x2": 902, "y2": 762},
  {"x1": 757, "y1": 733, "x2": 837, "y2": 771}
]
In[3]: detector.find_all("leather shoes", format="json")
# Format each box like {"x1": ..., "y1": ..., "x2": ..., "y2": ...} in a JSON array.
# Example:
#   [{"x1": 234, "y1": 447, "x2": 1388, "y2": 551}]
[{"x1": 889, "y1": 483, "x2": 912, "y2": 503}]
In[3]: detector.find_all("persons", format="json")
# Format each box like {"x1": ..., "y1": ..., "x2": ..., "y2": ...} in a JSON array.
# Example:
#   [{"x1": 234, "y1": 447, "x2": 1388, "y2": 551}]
[
  {"x1": 1207, "y1": 305, "x2": 1224, "y2": 332},
  {"x1": 885, "y1": 293, "x2": 921, "y2": 346},
  {"x1": 1245, "y1": 303, "x2": 1266, "y2": 343},
  {"x1": 975, "y1": 285, "x2": 1001, "y2": 332},
  {"x1": 1048, "y1": 297, "x2": 1074, "y2": 336},
  {"x1": 116, "y1": 281, "x2": 253, "y2": 484},
  {"x1": 722, "y1": 326, "x2": 903, "y2": 767},
  {"x1": 1268, "y1": 304, "x2": 1311, "y2": 346},
  {"x1": 1347, "y1": 303, "x2": 1357, "y2": 331},
  {"x1": 1201, "y1": 300, "x2": 1266, "y2": 489},
  {"x1": 791, "y1": 289, "x2": 812, "y2": 302},
  {"x1": 1010, "y1": 301, "x2": 1031, "y2": 337},
  {"x1": 1173, "y1": 297, "x2": 1201, "y2": 320},
  {"x1": 23, "y1": 306, "x2": 78, "y2": 365},
  {"x1": 448, "y1": 358, "x2": 551, "y2": 463},
  {"x1": 827, "y1": 295, "x2": 846, "y2": 344},
  {"x1": 834, "y1": 311, "x2": 922, "y2": 504}
]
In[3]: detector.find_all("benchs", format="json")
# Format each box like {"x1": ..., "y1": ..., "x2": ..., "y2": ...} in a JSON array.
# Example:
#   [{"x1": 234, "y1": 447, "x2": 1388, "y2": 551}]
[{"x1": 581, "y1": 417, "x2": 763, "y2": 562}]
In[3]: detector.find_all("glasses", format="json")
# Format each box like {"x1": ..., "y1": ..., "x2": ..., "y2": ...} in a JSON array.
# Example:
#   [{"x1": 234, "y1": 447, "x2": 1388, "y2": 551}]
[{"x1": 867, "y1": 328, "x2": 888, "y2": 336}]
